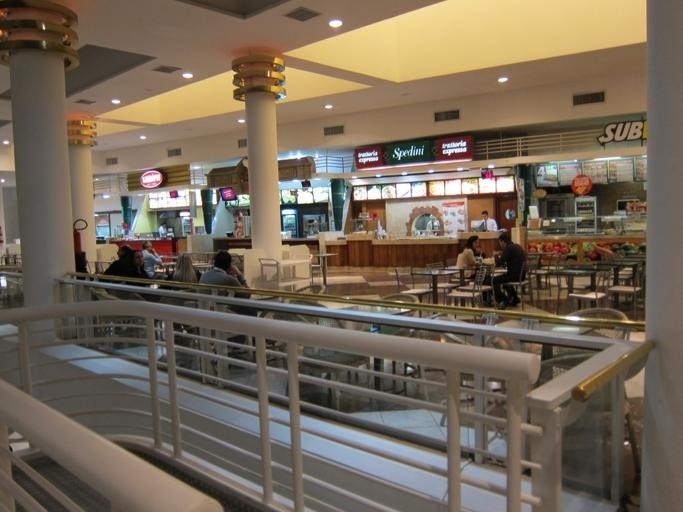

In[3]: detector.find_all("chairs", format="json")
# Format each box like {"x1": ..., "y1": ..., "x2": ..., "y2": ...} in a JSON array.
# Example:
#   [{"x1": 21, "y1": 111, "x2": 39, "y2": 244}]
[
  {"x1": 295, "y1": 284, "x2": 326, "y2": 303},
  {"x1": 440, "y1": 352, "x2": 641, "y2": 476},
  {"x1": 381, "y1": 251, "x2": 647, "y2": 337}
]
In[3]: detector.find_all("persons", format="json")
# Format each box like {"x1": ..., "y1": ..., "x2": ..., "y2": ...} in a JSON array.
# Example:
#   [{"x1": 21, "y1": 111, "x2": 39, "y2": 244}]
[
  {"x1": 104, "y1": 241, "x2": 251, "y2": 299},
  {"x1": 159, "y1": 223, "x2": 167, "y2": 237},
  {"x1": 456, "y1": 232, "x2": 524, "y2": 310},
  {"x1": 481, "y1": 211, "x2": 498, "y2": 232}
]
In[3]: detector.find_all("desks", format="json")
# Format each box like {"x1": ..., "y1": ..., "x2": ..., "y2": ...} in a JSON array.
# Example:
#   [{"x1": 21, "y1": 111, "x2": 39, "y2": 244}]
[
  {"x1": 160, "y1": 256, "x2": 177, "y2": 275},
  {"x1": 298, "y1": 252, "x2": 338, "y2": 286},
  {"x1": 250, "y1": 289, "x2": 281, "y2": 317}
]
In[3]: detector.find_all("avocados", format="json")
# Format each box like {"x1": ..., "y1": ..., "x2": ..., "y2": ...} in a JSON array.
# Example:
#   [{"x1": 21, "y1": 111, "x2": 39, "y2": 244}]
[{"x1": 611, "y1": 242, "x2": 646, "y2": 255}]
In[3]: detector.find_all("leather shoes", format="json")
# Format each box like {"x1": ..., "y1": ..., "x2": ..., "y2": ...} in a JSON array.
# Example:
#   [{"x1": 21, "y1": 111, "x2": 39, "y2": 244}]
[{"x1": 498, "y1": 296, "x2": 521, "y2": 309}]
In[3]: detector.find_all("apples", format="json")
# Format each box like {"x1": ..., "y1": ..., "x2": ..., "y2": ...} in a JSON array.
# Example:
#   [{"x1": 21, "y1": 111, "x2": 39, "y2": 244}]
[
  {"x1": 582, "y1": 240, "x2": 624, "y2": 260},
  {"x1": 528, "y1": 241, "x2": 577, "y2": 256}
]
